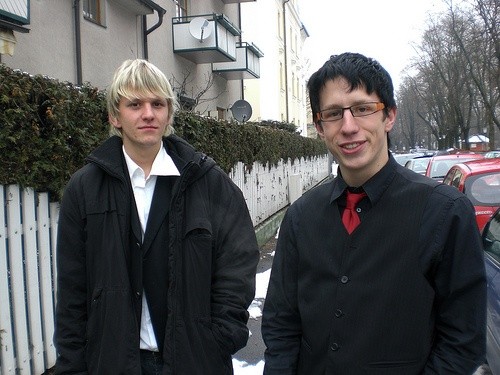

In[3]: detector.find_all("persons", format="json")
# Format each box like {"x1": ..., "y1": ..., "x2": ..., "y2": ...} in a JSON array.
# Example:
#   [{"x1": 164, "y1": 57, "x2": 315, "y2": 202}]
[
  {"x1": 261, "y1": 51, "x2": 487, "y2": 375},
  {"x1": 41, "y1": 59, "x2": 262, "y2": 375}
]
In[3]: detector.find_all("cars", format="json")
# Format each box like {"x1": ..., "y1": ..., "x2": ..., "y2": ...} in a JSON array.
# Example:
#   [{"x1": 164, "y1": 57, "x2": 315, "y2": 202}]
[
  {"x1": 441, "y1": 158, "x2": 500, "y2": 233},
  {"x1": 485, "y1": 151, "x2": 500, "y2": 158},
  {"x1": 391, "y1": 149, "x2": 449, "y2": 176},
  {"x1": 426, "y1": 152, "x2": 484, "y2": 184},
  {"x1": 480, "y1": 207, "x2": 500, "y2": 375}
]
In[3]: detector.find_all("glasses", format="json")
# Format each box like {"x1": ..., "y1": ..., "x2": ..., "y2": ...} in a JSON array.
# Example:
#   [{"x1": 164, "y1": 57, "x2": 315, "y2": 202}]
[{"x1": 317, "y1": 102, "x2": 385, "y2": 122}]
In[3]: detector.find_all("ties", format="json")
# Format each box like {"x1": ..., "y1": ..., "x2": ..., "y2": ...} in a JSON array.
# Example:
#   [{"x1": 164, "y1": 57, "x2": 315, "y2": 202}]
[{"x1": 342, "y1": 190, "x2": 367, "y2": 235}]
